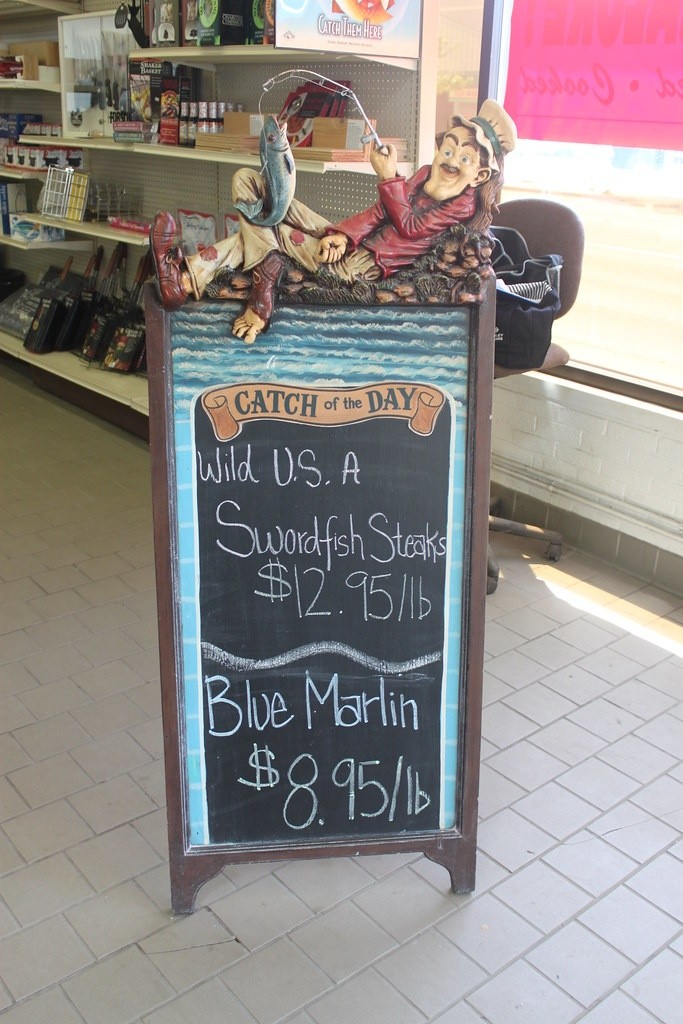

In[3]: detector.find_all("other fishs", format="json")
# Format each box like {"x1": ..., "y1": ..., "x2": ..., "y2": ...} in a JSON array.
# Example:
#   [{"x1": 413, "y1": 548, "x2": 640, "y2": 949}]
[{"x1": 232, "y1": 113, "x2": 296, "y2": 226}]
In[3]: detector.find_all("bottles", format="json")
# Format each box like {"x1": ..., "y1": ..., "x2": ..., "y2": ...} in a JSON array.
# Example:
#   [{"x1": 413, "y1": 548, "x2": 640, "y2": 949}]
[
  {"x1": 187, "y1": 100, "x2": 198, "y2": 147},
  {"x1": 195, "y1": 100, "x2": 209, "y2": 133},
  {"x1": 227, "y1": 103, "x2": 233, "y2": 112},
  {"x1": 236, "y1": 103, "x2": 244, "y2": 112},
  {"x1": 179, "y1": 100, "x2": 190, "y2": 146},
  {"x1": 216, "y1": 102, "x2": 226, "y2": 133},
  {"x1": 208, "y1": 100, "x2": 218, "y2": 134}
]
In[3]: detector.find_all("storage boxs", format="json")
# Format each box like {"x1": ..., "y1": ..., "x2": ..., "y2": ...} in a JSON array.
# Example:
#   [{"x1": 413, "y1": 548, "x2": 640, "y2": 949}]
[
  {"x1": 8, "y1": 41, "x2": 59, "y2": 66},
  {"x1": 1, "y1": 183, "x2": 31, "y2": 236},
  {"x1": 126, "y1": 0, "x2": 424, "y2": 122},
  {"x1": 9, "y1": 213, "x2": 67, "y2": 242}
]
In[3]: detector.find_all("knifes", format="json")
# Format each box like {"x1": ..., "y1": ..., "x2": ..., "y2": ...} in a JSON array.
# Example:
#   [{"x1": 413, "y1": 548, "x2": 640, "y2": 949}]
[{"x1": 95, "y1": 31, "x2": 126, "y2": 110}]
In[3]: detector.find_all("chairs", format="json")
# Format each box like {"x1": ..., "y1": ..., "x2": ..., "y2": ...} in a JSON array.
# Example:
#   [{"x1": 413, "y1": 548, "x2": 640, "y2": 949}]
[{"x1": 462, "y1": 198, "x2": 584, "y2": 595}]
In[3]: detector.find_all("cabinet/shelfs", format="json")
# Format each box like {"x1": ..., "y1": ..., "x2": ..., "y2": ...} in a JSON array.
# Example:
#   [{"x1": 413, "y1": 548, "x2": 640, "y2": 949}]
[{"x1": 0, "y1": 0, "x2": 424, "y2": 442}]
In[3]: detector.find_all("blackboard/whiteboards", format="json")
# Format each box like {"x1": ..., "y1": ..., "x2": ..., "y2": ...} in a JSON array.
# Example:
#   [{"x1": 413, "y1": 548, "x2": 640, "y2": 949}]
[{"x1": 141, "y1": 269, "x2": 496, "y2": 915}]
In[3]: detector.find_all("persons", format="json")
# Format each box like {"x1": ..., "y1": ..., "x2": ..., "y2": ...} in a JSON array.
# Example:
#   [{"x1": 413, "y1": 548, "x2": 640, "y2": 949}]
[{"x1": 149, "y1": 98, "x2": 518, "y2": 345}]
